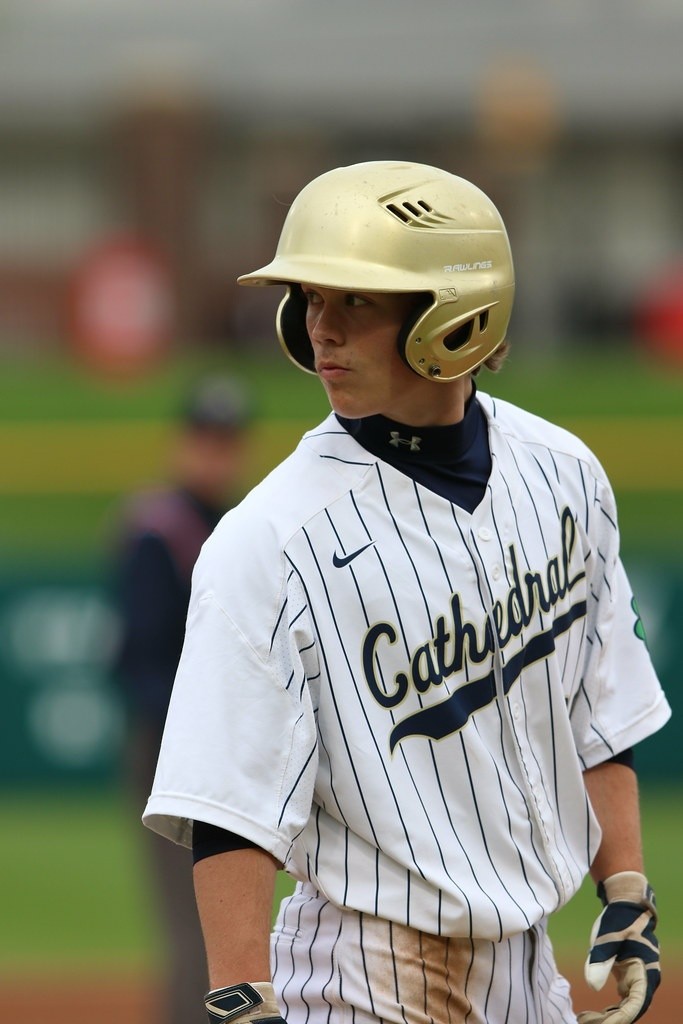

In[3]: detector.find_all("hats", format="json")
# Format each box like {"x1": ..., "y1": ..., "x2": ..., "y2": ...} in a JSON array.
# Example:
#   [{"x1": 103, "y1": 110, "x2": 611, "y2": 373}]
[{"x1": 171, "y1": 371, "x2": 260, "y2": 433}]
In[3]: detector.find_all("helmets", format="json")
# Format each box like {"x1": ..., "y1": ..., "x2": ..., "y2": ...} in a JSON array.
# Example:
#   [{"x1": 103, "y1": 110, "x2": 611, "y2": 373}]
[{"x1": 237, "y1": 159, "x2": 516, "y2": 383}]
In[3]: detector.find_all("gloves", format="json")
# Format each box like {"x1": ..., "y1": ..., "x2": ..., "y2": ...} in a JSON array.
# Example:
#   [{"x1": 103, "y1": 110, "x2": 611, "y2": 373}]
[
  {"x1": 204, "y1": 982, "x2": 289, "y2": 1024},
  {"x1": 576, "y1": 871, "x2": 662, "y2": 1024}
]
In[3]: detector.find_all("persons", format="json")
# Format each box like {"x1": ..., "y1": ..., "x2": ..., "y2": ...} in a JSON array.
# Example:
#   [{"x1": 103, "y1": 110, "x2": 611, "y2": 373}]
[
  {"x1": 141, "y1": 160, "x2": 672, "y2": 1024},
  {"x1": 109, "y1": 377, "x2": 253, "y2": 1024}
]
